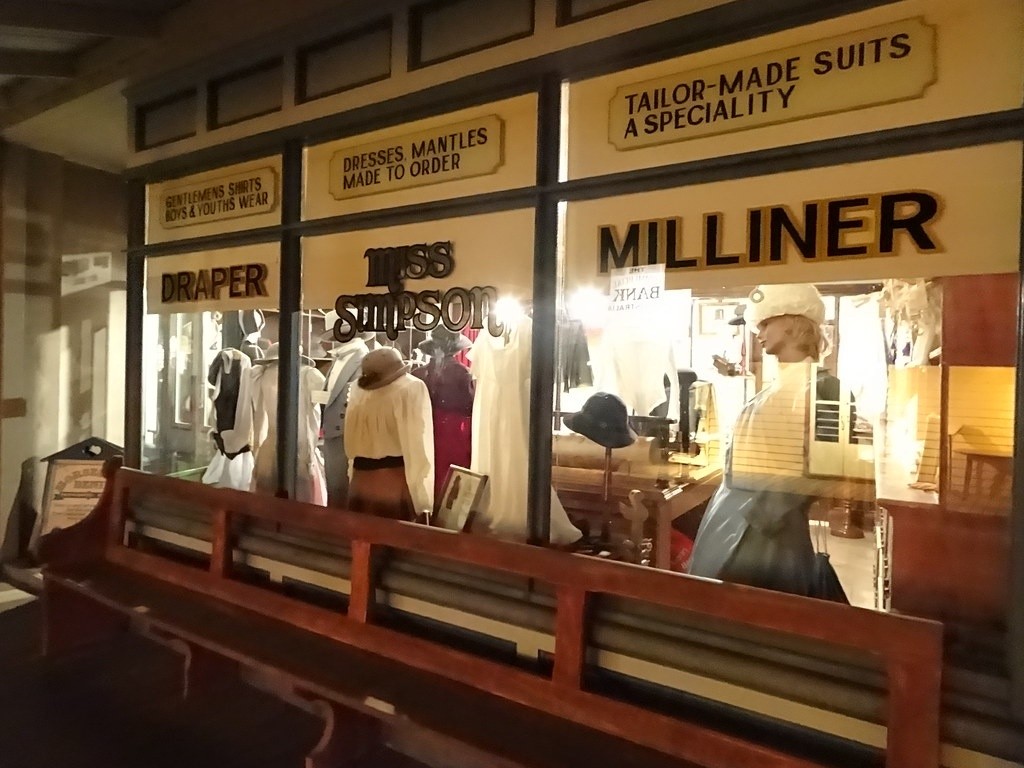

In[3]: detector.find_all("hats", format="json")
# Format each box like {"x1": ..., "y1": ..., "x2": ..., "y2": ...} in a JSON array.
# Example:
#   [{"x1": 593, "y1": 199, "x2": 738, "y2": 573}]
[
  {"x1": 357, "y1": 346, "x2": 411, "y2": 390},
  {"x1": 417, "y1": 324, "x2": 472, "y2": 358},
  {"x1": 254, "y1": 342, "x2": 317, "y2": 367},
  {"x1": 320, "y1": 307, "x2": 377, "y2": 342},
  {"x1": 563, "y1": 392, "x2": 638, "y2": 448},
  {"x1": 239, "y1": 308, "x2": 265, "y2": 342},
  {"x1": 743, "y1": 283, "x2": 826, "y2": 335}
]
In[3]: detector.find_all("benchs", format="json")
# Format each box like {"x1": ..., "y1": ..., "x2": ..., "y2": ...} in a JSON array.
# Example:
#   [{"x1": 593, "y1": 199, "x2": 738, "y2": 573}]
[{"x1": 39, "y1": 465, "x2": 946, "y2": 768}]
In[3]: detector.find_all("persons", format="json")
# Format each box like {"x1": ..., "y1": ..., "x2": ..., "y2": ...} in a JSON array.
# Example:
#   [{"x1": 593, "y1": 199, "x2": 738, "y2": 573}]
[
  {"x1": 409, "y1": 324, "x2": 477, "y2": 496},
  {"x1": 201, "y1": 309, "x2": 376, "y2": 507},
  {"x1": 344, "y1": 347, "x2": 435, "y2": 526},
  {"x1": 688, "y1": 283, "x2": 857, "y2": 607}
]
